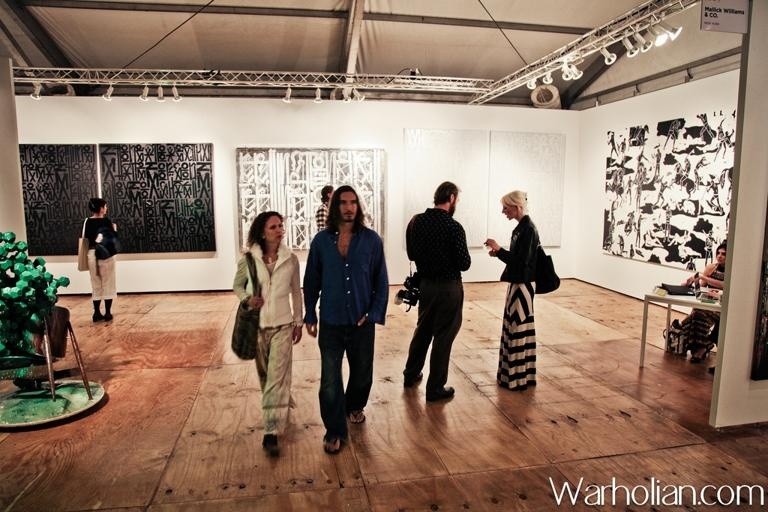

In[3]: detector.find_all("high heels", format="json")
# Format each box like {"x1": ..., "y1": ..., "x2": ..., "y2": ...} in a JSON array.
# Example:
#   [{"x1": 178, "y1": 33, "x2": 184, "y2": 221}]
[
  {"x1": 690, "y1": 344, "x2": 714, "y2": 362},
  {"x1": 709, "y1": 368, "x2": 715, "y2": 373}
]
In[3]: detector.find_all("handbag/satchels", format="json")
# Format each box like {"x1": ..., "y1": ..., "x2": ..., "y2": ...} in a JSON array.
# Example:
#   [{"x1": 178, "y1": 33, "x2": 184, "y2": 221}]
[
  {"x1": 535, "y1": 247, "x2": 560, "y2": 294},
  {"x1": 663, "y1": 319, "x2": 689, "y2": 357},
  {"x1": 95, "y1": 238, "x2": 123, "y2": 260},
  {"x1": 232, "y1": 302, "x2": 260, "y2": 359},
  {"x1": 78, "y1": 237, "x2": 89, "y2": 271}
]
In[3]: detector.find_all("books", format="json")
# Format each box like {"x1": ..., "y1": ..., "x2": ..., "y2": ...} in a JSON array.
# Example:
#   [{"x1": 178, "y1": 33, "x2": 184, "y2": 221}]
[{"x1": 662, "y1": 283, "x2": 695, "y2": 295}]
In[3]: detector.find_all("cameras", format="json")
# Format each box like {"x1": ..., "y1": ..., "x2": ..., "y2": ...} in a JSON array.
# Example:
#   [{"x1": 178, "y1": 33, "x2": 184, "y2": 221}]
[{"x1": 397, "y1": 272, "x2": 419, "y2": 306}]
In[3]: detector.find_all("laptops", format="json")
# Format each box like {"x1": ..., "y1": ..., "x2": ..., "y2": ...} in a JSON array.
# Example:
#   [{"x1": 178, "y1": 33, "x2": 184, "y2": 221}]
[{"x1": 694, "y1": 272, "x2": 723, "y2": 302}]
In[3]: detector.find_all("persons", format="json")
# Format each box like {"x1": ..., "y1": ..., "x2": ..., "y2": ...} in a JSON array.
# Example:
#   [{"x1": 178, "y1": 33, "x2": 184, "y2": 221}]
[
  {"x1": 484, "y1": 191, "x2": 540, "y2": 389},
  {"x1": 81, "y1": 197, "x2": 118, "y2": 322},
  {"x1": 316, "y1": 186, "x2": 333, "y2": 230},
  {"x1": 302, "y1": 185, "x2": 389, "y2": 454},
  {"x1": 402, "y1": 182, "x2": 471, "y2": 402},
  {"x1": 607, "y1": 114, "x2": 734, "y2": 270},
  {"x1": 232, "y1": 211, "x2": 302, "y2": 456},
  {"x1": 681, "y1": 239, "x2": 727, "y2": 364}
]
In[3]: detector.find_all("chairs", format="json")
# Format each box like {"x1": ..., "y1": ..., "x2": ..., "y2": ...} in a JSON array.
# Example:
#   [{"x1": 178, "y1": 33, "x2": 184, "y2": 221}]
[{"x1": 0, "y1": 307, "x2": 92, "y2": 400}]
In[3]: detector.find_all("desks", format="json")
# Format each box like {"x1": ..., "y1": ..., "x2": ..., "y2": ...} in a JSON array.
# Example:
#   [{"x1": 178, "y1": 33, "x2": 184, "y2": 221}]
[{"x1": 640, "y1": 294, "x2": 722, "y2": 367}]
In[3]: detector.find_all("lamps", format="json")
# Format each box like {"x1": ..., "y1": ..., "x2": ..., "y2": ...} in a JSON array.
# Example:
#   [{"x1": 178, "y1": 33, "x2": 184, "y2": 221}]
[
  {"x1": 467, "y1": 0, "x2": 702, "y2": 104},
  {"x1": 9, "y1": 57, "x2": 496, "y2": 104}
]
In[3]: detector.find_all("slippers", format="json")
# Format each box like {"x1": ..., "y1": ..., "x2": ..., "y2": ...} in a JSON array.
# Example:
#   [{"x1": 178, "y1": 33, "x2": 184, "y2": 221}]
[
  {"x1": 324, "y1": 439, "x2": 340, "y2": 455},
  {"x1": 349, "y1": 411, "x2": 366, "y2": 424}
]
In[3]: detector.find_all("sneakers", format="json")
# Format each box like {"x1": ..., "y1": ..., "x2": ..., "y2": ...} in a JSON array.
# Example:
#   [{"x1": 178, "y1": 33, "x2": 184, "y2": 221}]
[
  {"x1": 103, "y1": 314, "x2": 112, "y2": 321},
  {"x1": 427, "y1": 387, "x2": 454, "y2": 401},
  {"x1": 404, "y1": 372, "x2": 422, "y2": 386},
  {"x1": 92, "y1": 312, "x2": 102, "y2": 322},
  {"x1": 263, "y1": 435, "x2": 280, "y2": 457}
]
